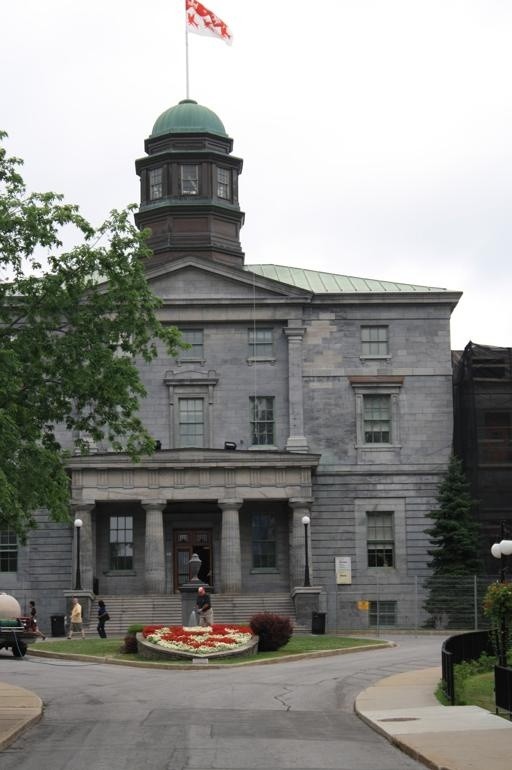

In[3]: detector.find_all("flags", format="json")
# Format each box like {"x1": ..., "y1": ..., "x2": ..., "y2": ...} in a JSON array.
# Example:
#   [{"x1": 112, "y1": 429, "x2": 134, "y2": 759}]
[{"x1": 185, "y1": 0, "x2": 232, "y2": 44}]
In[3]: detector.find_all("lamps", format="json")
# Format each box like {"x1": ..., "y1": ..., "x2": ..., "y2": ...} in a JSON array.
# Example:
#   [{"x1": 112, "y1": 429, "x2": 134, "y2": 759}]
[
  {"x1": 156, "y1": 440, "x2": 161, "y2": 449},
  {"x1": 225, "y1": 442, "x2": 236, "y2": 450}
]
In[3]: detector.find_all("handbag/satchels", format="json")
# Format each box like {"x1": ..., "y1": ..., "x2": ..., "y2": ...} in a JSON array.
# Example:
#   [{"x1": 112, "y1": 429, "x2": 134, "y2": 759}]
[{"x1": 99, "y1": 612, "x2": 109, "y2": 623}]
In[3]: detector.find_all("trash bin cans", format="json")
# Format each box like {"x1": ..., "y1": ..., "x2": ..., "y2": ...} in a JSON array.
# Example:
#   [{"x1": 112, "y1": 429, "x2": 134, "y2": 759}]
[
  {"x1": 93, "y1": 578, "x2": 98, "y2": 595},
  {"x1": 312, "y1": 611, "x2": 325, "y2": 634},
  {"x1": 50, "y1": 615, "x2": 68, "y2": 637}
]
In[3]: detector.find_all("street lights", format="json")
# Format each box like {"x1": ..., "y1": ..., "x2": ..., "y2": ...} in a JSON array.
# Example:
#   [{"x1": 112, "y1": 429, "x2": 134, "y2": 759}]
[
  {"x1": 491, "y1": 539, "x2": 512, "y2": 666},
  {"x1": 73, "y1": 519, "x2": 84, "y2": 588},
  {"x1": 300, "y1": 516, "x2": 311, "y2": 586}
]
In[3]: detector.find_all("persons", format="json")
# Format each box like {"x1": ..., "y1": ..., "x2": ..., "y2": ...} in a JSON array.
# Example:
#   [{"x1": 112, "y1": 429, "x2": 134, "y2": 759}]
[
  {"x1": 67, "y1": 598, "x2": 87, "y2": 639},
  {"x1": 29, "y1": 600, "x2": 46, "y2": 641},
  {"x1": 96, "y1": 599, "x2": 107, "y2": 638},
  {"x1": 195, "y1": 586, "x2": 214, "y2": 625}
]
